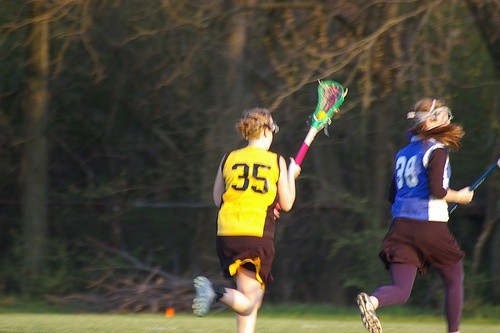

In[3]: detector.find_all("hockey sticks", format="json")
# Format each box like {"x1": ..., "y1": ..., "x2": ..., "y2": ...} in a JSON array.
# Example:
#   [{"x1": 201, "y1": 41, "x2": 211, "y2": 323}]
[
  {"x1": 446, "y1": 158, "x2": 500, "y2": 218},
  {"x1": 272, "y1": 77, "x2": 348, "y2": 218}
]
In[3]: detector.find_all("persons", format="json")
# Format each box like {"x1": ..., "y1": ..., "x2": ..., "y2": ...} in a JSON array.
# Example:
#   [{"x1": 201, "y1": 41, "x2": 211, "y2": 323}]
[
  {"x1": 353, "y1": 96, "x2": 473, "y2": 332},
  {"x1": 191, "y1": 106, "x2": 302, "y2": 333}
]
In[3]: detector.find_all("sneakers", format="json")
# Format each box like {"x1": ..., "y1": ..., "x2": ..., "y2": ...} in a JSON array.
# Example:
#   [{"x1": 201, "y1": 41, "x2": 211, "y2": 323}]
[
  {"x1": 192, "y1": 275, "x2": 215, "y2": 317},
  {"x1": 356, "y1": 292, "x2": 382, "y2": 333}
]
